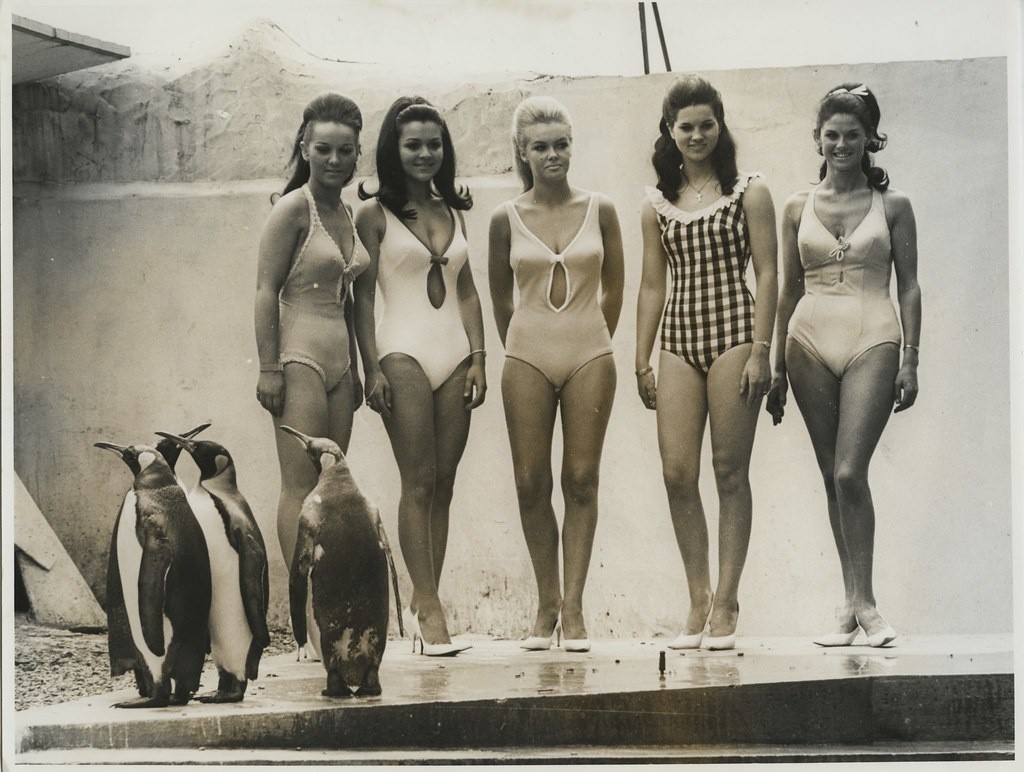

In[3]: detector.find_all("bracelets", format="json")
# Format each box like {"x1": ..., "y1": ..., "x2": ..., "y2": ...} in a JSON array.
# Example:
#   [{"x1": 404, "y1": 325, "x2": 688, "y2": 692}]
[
  {"x1": 471, "y1": 349, "x2": 487, "y2": 358},
  {"x1": 635, "y1": 367, "x2": 653, "y2": 376},
  {"x1": 903, "y1": 344, "x2": 919, "y2": 353},
  {"x1": 753, "y1": 341, "x2": 771, "y2": 348},
  {"x1": 260, "y1": 363, "x2": 283, "y2": 372}
]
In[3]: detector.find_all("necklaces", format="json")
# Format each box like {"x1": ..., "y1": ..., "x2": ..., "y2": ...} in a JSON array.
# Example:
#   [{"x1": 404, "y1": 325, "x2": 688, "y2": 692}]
[{"x1": 685, "y1": 172, "x2": 714, "y2": 202}]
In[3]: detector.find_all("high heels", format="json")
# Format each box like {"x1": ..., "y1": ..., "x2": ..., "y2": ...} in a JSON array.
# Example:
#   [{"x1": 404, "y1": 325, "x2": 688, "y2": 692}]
[
  {"x1": 403, "y1": 606, "x2": 474, "y2": 653},
  {"x1": 286, "y1": 615, "x2": 322, "y2": 663},
  {"x1": 558, "y1": 614, "x2": 590, "y2": 653},
  {"x1": 519, "y1": 607, "x2": 562, "y2": 650},
  {"x1": 406, "y1": 609, "x2": 463, "y2": 656}
]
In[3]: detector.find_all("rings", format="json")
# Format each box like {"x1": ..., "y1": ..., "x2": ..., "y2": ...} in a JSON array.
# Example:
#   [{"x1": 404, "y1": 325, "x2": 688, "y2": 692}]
[
  {"x1": 366, "y1": 402, "x2": 371, "y2": 406},
  {"x1": 763, "y1": 391, "x2": 767, "y2": 394}
]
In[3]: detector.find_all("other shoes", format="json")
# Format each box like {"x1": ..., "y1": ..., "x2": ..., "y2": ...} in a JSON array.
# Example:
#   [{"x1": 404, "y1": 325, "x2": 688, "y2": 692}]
[
  {"x1": 668, "y1": 591, "x2": 715, "y2": 648},
  {"x1": 856, "y1": 609, "x2": 898, "y2": 648},
  {"x1": 701, "y1": 599, "x2": 740, "y2": 650},
  {"x1": 813, "y1": 622, "x2": 862, "y2": 647}
]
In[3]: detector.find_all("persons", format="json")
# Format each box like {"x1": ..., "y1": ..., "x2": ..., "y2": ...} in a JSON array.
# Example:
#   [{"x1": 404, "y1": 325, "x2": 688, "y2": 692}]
[
  {"x1": 488, "y1": 95, "x2": 625, "y2": 651},
  {"x1": 636, "y1": 74, "x2": 777, "y2": 651},
  {"x1": 766, "y1": 84, "x2": 922, "y2": 649},
  {"x1": 354, "y1": 95, "x2": 487, "y2": 656},
  {"x1": 254, "y1": 93, "x2": 371, "y2": 661}
]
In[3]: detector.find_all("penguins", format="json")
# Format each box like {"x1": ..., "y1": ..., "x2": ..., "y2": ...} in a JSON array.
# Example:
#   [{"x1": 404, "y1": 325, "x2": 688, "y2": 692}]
[{"x1": 85, "y1": 422, "x2": 404, "y2": 709}]
